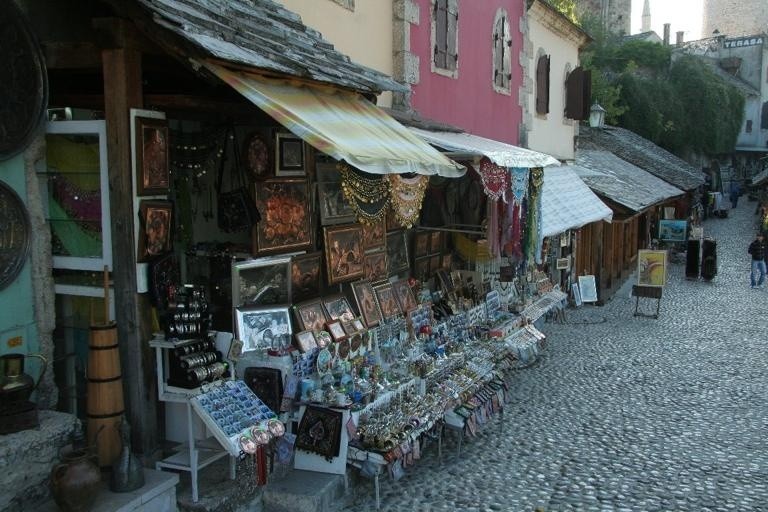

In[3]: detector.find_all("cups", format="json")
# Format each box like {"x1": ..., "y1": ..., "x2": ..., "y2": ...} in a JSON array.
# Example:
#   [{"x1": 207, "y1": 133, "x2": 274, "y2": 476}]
[
  {"x1": 336, "y1": 392, "x2": 346, "y2": 402},
  {"x1": 312, "y1": 389, "x2": 325, "y2": 398}
]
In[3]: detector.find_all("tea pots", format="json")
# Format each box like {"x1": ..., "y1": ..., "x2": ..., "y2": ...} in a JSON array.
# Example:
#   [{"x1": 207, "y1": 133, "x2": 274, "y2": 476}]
[{"x1": 0, "y1": 353, "x2": 46, "y2": 411}]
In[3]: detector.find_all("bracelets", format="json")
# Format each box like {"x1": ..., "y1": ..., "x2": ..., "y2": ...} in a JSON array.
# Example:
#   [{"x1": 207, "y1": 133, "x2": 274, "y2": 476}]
[{"x1": 168, "y1": 311, "x2": 225, "y2": 382}]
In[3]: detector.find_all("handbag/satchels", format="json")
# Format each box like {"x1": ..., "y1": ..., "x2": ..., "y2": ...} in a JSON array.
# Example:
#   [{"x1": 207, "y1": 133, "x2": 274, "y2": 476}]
[
  {"x1": 217, "y1": 187, "x2": 261, "y2": 233},
  {"x1": 143, "y1": 251, "x2": 180, "y2": 307},
  {"x1": 170, "y1": 132, "x2": 222, "y2": 190}
]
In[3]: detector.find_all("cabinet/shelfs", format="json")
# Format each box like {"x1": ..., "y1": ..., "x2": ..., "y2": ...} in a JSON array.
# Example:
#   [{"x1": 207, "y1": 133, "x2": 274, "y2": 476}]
[
  {"x1": 149, "y1": 330, "x2": 236, "y2": 503},
  {"x1": 237, "y1": 351, "x2": 508, "y2": 509}
]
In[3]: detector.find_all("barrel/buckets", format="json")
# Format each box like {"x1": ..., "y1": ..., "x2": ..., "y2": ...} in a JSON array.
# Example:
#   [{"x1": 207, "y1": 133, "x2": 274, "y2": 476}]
[{"x1": 85, "y1": 320, "x2": 125, "y2": 467}]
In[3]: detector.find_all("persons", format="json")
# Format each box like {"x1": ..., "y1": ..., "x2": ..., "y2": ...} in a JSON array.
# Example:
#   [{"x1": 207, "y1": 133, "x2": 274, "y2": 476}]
[
  {"x1": 748, "y1": 200, "x2": 768, "y2": 290},
  {"x1": 729, "y1": 174, "x2": 741, "y2": 209}
]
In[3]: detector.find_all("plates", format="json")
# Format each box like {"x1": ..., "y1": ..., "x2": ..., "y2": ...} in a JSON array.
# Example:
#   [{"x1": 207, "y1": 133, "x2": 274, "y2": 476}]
[{"x1": 317, "y1": 350, "x2": 332, "y2": 376}]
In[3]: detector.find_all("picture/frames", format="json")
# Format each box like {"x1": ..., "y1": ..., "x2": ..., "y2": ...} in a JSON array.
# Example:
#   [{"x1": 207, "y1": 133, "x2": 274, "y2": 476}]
[
  {"x1": 135, "y1": 116, "x2": 176, "y2": 263},
  {"x1": 230, "y1": 131, "x2": 478, "y2": 353}
]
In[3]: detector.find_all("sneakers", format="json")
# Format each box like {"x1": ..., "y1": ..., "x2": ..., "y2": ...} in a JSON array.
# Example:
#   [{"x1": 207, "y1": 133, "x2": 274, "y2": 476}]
[{"x1": 753, "y1": 284, "x2": 764, "y2": 289}]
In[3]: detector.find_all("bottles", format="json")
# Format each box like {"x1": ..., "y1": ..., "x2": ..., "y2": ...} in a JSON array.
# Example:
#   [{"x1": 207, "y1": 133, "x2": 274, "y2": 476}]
[
  {"x1": 110, "y1": 415, "x2": 145, "y2": 493},
  {"x1": 53, "y1": 451, "x2": 102, "y2": 511}
]
